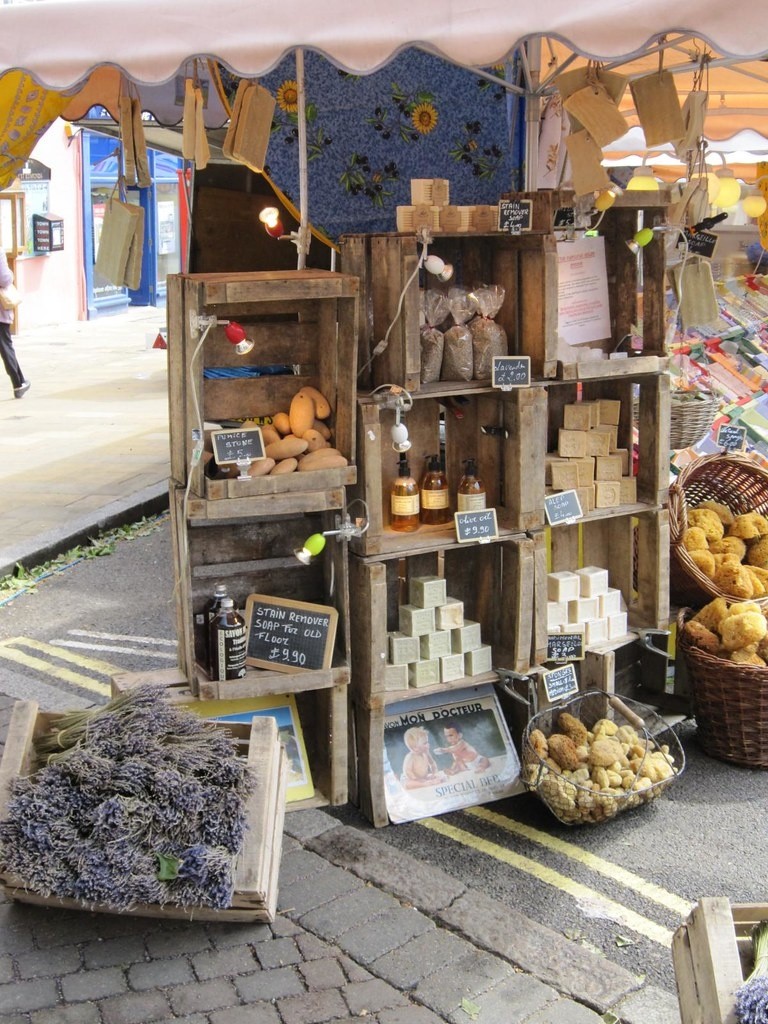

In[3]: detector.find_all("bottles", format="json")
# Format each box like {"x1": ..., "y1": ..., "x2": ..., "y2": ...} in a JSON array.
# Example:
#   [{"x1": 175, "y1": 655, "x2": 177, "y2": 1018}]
[
  {"x1": 457, "y1": 458, "x2": 486, "y2": 511},
  {"x1": 391, "y1": 460, "x2": 420, "y2": 532},
  {"x1": 421, "y1": 455, "x2": 450, "y2": 525},
  {"x1": 209, "y1": 585, "x2": 246, "y2": 681}
]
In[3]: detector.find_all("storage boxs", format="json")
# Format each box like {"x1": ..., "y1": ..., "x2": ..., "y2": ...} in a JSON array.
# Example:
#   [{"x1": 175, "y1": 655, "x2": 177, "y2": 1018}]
[
  {"x1": 633, "y1": 272, "x2": 768, "y2": 475},
  {"x1": 528, "y1": 508, "x2": 670, "y2": 668},
  {"x1": 0, "y1": 700, "x2": 289, "y2": 924},
  {"x1": 348, "y1": 533, "x2": 534, "y2": 709},
  {"x1": 337, "y1": 230, "x2": 557, "y2": 393},
  {"x1": 347, "y1": 668, "x2": 539, "y2": 829},
  {"x1": 526, "y1": 372, "x2": 670, "y2": 533},
  {"x1": 166, "y1": 269, "x2": 360, "y2": 501},
  {"x1": 499, "y1": 188, "x2": 671, "y2": 383},
  {"x1": 347, "y1": 391, "x2": 531, "y2": 558},
  {"x1": 671, "y1": 896, "x2": 768, "y2": 1024},
  {"x1": 546, "y1": 640, "x2": 694, "y2": 740},
  {"x1": 167, "y1": 477, "x2": 353, "y2": 701},
  {"x1": 111, "y1": 666, "x2": 348, "y2": 813}
]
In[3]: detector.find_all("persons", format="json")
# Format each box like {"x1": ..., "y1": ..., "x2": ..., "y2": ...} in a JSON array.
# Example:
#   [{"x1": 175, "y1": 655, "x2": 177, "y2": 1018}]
[
  {"x1": 0, "y1": 245, "x2": 30, "y2": 399},
  {"x1": 400, "y1": 722, "x2": 490, "y2": 790}
]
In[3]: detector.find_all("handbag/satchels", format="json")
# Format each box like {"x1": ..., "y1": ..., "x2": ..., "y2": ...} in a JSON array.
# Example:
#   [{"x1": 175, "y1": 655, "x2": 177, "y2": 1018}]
[{"x1": 1, "y1": 284, "x2": 22, "y2": 309}]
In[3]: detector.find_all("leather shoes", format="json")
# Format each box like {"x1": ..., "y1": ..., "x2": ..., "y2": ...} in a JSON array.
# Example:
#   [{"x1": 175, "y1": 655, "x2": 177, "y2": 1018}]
[{"x1": 14, "y1": 380, "x2": 31, "y2": 399}]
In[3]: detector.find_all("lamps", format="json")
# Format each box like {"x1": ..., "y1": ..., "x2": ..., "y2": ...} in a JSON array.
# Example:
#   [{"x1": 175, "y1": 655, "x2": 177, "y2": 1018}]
[
  {"x1": 625, "y1": 151, "x2": 678, "y2": 190},
  {"x1": 742, "y1": 174, "x2": 768, "y2": 217},
  {"x1": 705, "y1": 150, "x2": 741, "y2": 209},
  {"x1": 691, "y1": 163, "x2": 721, "y2": 204}
]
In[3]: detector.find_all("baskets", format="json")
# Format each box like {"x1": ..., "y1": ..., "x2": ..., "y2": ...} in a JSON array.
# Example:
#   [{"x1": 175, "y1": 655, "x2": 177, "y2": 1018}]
[
  {"x1": 521, "y1": 690, "x2": 686, "y2": 826},
  {"x1": 632, "y1": 389, "x2": 724, "y2": 450},
  {"x1": 669, "y1": 453, "x2": 768, "y2": 605},
  {"x1": 678, "y1": 606, "x2": 768, "y2": 768}
]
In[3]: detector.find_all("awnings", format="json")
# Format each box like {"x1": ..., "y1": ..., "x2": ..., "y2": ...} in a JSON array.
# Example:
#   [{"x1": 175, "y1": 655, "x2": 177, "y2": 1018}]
[{"x1": 0, "y1": 4, "x2": 768, "y2": 195}]
